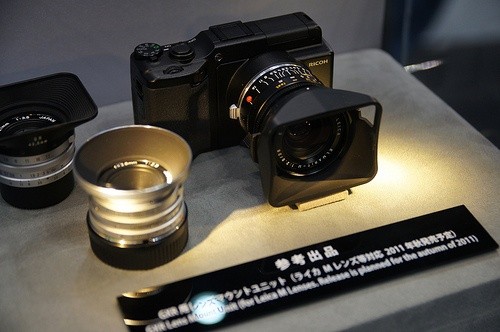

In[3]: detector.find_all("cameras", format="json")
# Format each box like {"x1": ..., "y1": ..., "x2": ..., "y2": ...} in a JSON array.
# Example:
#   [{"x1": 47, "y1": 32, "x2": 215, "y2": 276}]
[{"x1": 129, "y1": 11, "x2": 383, "y2": 208}]
